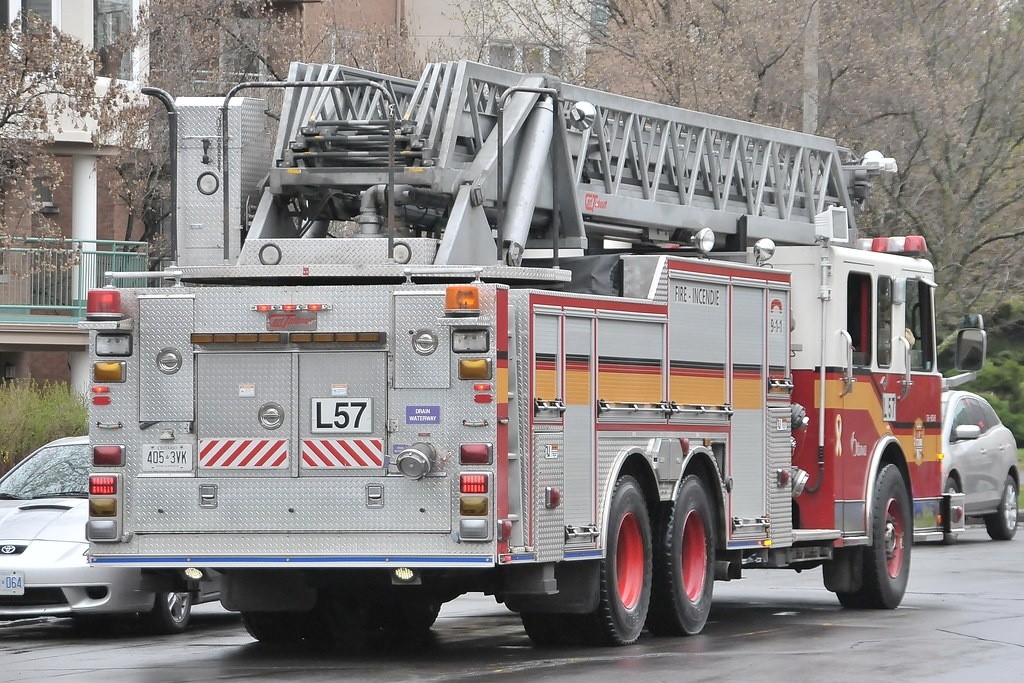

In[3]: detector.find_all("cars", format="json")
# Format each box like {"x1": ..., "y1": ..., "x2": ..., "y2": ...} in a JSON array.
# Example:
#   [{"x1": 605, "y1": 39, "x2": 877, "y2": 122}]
[
  {"x1": 0, "y1": 436, "x2": 220, "y2": 636},
  {"x1": 913, "y1": 389, "x2": 1020, "y2": 543}
]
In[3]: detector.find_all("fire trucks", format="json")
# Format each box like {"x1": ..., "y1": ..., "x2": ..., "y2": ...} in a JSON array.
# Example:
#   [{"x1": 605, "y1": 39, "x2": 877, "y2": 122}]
[{"x1": 80, "y1": 59, "x2": 989, "y2": 648}]
